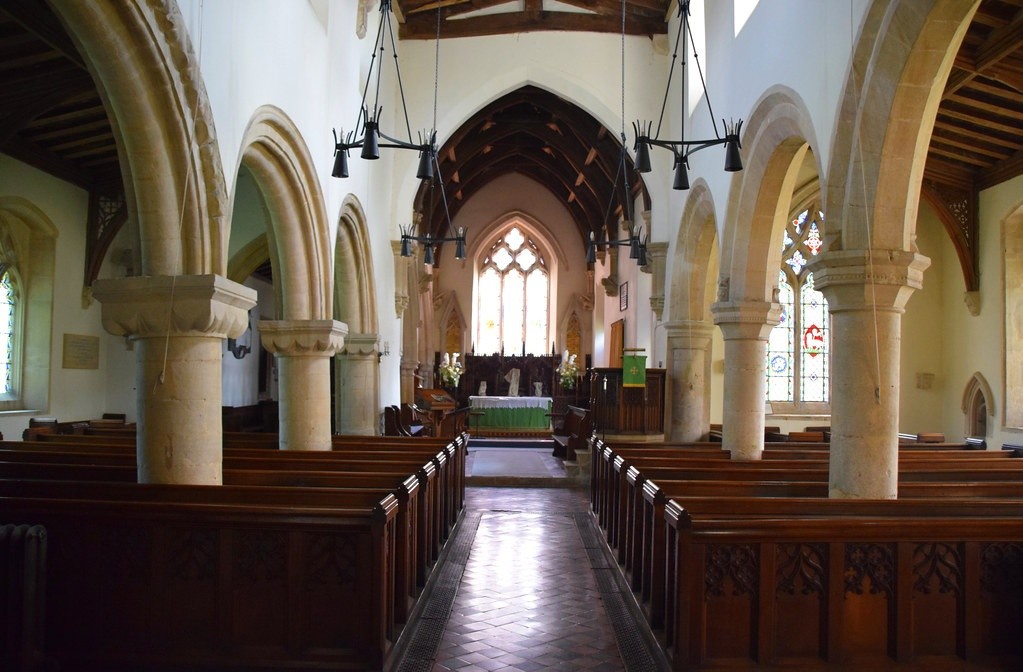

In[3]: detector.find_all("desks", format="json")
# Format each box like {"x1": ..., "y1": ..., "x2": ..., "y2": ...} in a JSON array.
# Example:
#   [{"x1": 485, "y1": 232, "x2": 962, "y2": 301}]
[{"x1": 469, "y1": 396, "x2": 553, "y2": 429}]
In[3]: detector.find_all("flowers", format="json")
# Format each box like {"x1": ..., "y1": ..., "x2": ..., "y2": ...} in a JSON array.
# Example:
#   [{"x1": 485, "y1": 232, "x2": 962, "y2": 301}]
[
  {"x1": 559, "y1": 363, "x2": 577, "y2": 390},
  {"x1": 442, "y1": 366, "x2": 463, "y2": 388}
]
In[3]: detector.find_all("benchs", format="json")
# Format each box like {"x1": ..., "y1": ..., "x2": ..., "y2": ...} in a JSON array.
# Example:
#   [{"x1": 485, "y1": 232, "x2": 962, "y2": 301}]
[
  {"x1": 0, "y1": 410, "x2": 470, "y2": 672},
  {"x1": 594, "y1": 439, "x2": 1022, "y2": 671},
  {"x1": 545, "y1": 405, "x2": 591, "y2": 461}
]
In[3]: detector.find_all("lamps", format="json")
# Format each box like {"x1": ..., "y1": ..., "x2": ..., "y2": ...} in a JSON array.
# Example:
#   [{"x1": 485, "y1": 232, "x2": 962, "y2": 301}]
[
  {"x1": 332, "y1": 0, "x2": 469, "y2": 265},
  {"x1": 586, "y1": 1, "x2": 743, "y2": 265}
]
